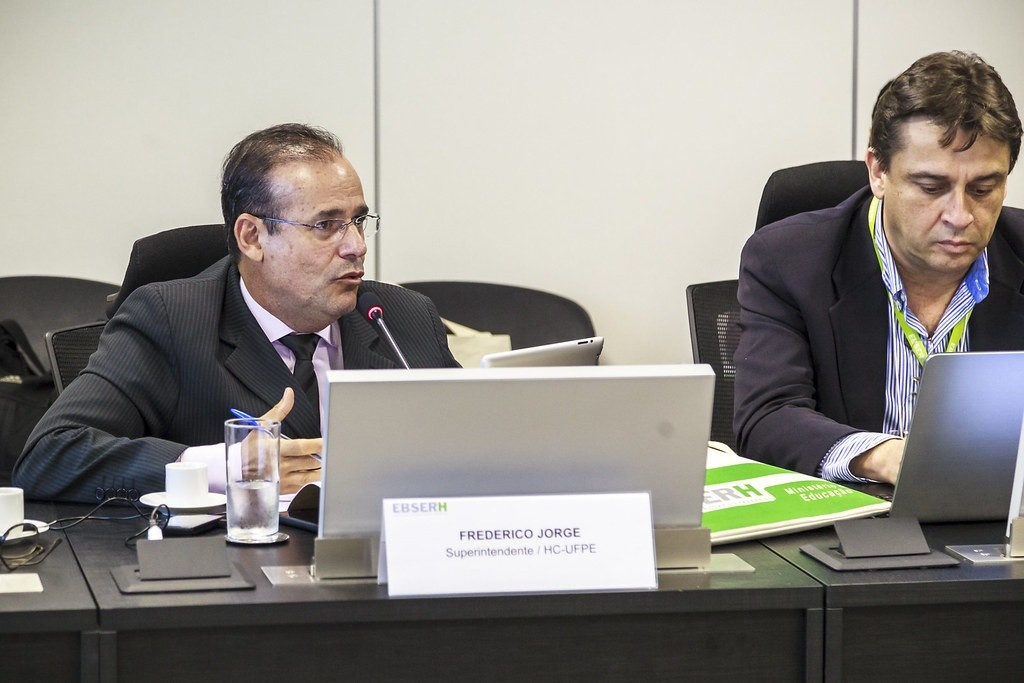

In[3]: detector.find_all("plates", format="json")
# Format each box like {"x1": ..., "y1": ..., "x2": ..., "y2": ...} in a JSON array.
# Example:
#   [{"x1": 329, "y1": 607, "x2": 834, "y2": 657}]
[
  {"x1": 138, "y1": 491, "x2": 227, "y2": 517},
  {"x1": 0, "y1": 519, "x2": 50, "y2": 547}
]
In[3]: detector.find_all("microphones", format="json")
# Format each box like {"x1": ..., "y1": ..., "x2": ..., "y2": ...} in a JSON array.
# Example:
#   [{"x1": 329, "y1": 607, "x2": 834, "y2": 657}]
[{"x1": 359, "y1": 291, "x2": 413, "y2": 371}]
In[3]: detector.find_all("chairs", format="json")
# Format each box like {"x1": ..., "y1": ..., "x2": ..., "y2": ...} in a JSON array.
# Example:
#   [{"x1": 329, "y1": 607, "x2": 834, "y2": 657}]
[
  {"x1": 44, "y1": 224, "x2": 229, "y2": 400},
  {"x1": 397, "y1": 281, "x2": 597, "y2": 349},
  {"x1": 685, "y1": 159, "x2": 870, "y2": 457},
  {"x1": 0, "y1": 276, "x2": 123, "y2": 379}
]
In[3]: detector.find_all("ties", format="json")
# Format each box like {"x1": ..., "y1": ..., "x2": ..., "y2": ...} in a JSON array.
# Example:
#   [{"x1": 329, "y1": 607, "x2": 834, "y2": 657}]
[{"x1": 286, "y1": 335, "x2": 324, "y2": 430}]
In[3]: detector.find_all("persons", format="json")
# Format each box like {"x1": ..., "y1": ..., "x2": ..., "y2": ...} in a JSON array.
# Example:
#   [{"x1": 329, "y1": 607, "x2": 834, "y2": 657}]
[
  {"x1": 13, "y1": 124, "x2": 463, "y2": 503},
  {"x1": 734, "y1": 51, "x2": 1024, "y2": 489}
]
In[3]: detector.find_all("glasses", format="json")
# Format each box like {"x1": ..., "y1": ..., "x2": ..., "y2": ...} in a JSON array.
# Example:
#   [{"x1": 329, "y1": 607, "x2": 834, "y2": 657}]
[{"x1": 259, "y1": 212, "x2": 380, "y2": 242}]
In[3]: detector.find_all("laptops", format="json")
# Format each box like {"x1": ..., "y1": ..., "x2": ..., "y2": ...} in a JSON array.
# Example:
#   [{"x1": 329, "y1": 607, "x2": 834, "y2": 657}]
[
  {"x1": 834, "y1": 351, "x2": 1024, "y2": 523},
  {"x1": 484, "y1": 337, "x2": 602, "y2": 368}
]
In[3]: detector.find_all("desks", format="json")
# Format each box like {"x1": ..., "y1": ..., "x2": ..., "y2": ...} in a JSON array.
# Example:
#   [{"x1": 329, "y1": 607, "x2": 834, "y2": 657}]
[{"x1": 0, "y1": 478, "x2": 1023, "y2": 682}]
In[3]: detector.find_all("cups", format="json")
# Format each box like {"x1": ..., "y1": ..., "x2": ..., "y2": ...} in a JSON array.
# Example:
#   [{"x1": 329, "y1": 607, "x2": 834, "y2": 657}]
[
  {"x1": 223, "y1": 416, "x2": 282, "y2": 542},
  {"x1": 0, "y1": 487, "x2": 24, "y2": 535},
  {"x1": 164, "y1": 461, "x2": 210, "y2": 507}
]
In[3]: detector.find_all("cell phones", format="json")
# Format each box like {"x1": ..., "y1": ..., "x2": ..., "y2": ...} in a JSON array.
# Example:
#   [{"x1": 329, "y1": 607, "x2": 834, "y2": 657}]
[{"x1": 165, "y1": 511, "x2": 222, "y2": 536}]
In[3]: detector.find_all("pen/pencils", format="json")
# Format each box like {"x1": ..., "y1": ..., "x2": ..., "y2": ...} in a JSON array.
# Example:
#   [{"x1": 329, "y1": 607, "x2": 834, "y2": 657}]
[{"x1": 232, "y1": 408, "x2": 321, "y2": 463}]
